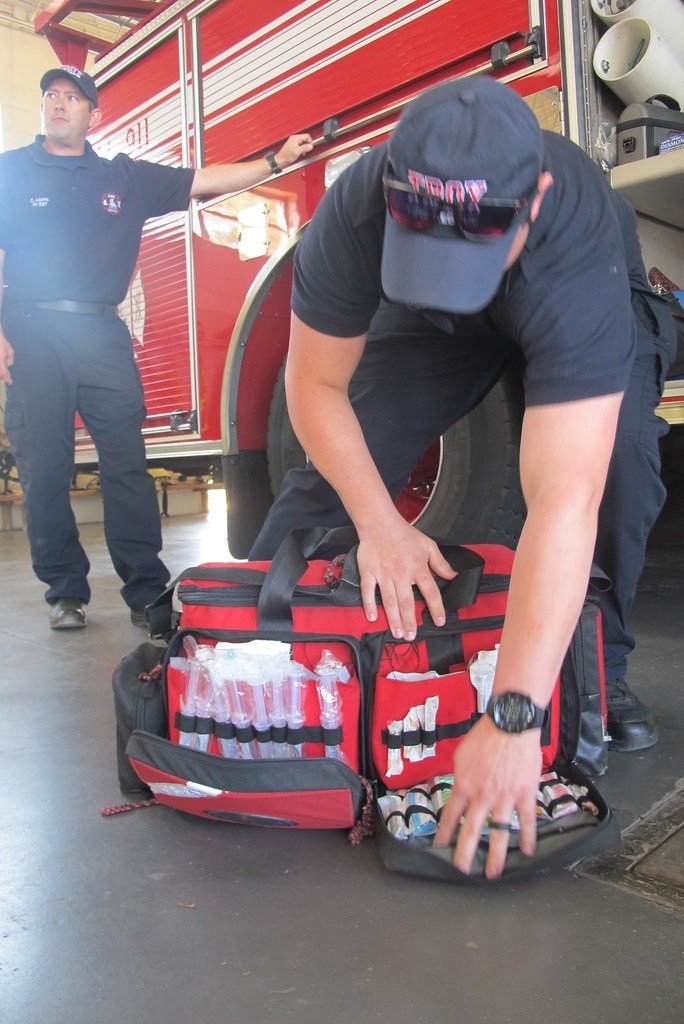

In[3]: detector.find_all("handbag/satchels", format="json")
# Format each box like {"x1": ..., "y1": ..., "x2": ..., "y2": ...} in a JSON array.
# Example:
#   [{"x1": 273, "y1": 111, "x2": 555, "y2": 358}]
[{"x1": 113, "y1": 525, "x2": 613, "y2": 886}]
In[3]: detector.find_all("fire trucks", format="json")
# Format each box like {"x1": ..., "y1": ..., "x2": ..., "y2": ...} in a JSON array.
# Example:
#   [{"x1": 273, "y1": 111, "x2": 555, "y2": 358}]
[{"x1": 34, "y1": 0, "x2": 684, "y2": 550}]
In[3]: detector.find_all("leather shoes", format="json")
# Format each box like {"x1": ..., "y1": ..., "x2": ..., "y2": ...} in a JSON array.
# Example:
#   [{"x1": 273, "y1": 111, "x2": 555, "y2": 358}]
[
  {"x1": 131, "y1": 610, "x2": 182, "y2": 629},
  {"x1": 600, "y1": 682, "x2": 658, "y2": 755},
  {"x1": 49, "y1": 600, "x2": 87, "y2": 629}
]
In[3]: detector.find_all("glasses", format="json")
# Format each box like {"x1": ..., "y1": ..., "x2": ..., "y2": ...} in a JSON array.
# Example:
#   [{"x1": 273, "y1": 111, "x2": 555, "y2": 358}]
[{"x1": 383, "y1": 159, "x2": 540, "y2": 239}]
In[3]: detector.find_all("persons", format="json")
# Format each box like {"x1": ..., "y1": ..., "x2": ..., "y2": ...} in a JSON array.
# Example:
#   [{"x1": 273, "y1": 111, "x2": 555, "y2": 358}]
[
  {"x1": 249, "y1": 75, "x2": 677, "y2": 879},
  {"x1": 1, "y1": 65, "x2": 313, "y2": 630}
]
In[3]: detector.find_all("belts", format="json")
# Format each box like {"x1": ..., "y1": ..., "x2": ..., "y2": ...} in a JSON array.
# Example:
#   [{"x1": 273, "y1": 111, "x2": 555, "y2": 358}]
[{"x1": 36, "y1": 300, "x2": 118, "y2": 317}]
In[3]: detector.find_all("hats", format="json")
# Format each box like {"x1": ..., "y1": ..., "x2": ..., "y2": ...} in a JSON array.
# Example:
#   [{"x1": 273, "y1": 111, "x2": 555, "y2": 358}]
[
  {"x1": 383, "y1": 76, "x2": 545, "y2": 314},
  {"x1": 39, "y1": 65, "x2": 97, "y2": 107}
]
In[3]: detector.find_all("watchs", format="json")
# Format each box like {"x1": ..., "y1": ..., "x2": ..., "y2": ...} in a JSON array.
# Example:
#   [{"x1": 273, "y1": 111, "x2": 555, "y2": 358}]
[
  {"x1": 264, "y1": 151, "x2": 282, "y2": 174},
  {"x1": 486, "y1": 690, "x2": 549, "y2": 733}
]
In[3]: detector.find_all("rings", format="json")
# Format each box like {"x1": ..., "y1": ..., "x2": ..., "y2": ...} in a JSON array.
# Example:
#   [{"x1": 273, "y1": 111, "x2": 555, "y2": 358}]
[{"x1": 489, "y1": 823, "x2": 511, "y2": 830}]
